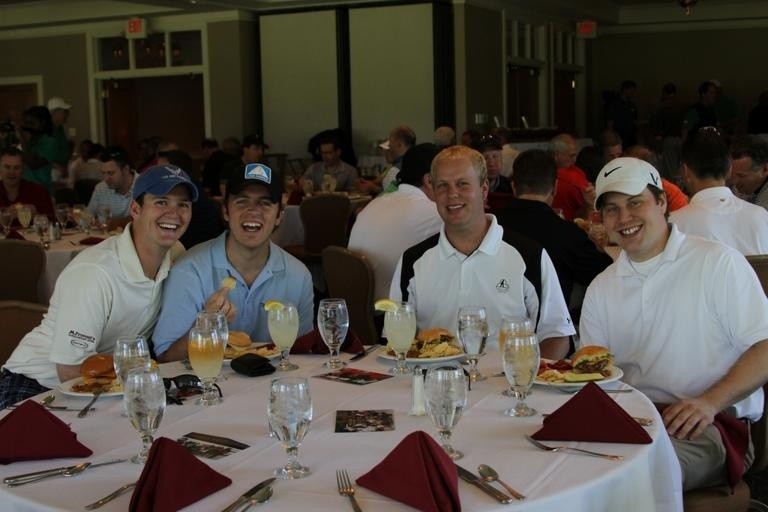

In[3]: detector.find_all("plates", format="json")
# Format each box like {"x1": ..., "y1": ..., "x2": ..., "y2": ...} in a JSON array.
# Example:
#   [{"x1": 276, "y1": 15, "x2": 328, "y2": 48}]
[
  {"x1": 222, "y1": 342, "x2": 281, "y2": 365},
  {"x1": 379, "y1": 349, "x2": 466, "y2": 363},
  {"x1": 534, "y1": 360, "x2": 624, "y2": 386},
  {"x1": 57, "y1": 375, "x2": 124, "y2": 398}
]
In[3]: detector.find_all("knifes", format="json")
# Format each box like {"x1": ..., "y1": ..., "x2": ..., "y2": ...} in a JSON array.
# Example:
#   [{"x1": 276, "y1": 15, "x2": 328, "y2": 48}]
[
  {"x1": 454, "y1": 462, "x2": 513, "y2": 505},
  {"x1": 542, "y1": 413, "x2": 652, "y2": 425},
  {"x1": 350, "y1": 343, "x2": 382, "y2": 361},
  {"x1": 4, "y1": 458, "x2": 126, "y2": 483},
  {"x1": 7, "y1": 405, "x2": 97, "y2": 411},
  {"x1": 223, "y1": 476, "x2": 275, "y2": 511}
]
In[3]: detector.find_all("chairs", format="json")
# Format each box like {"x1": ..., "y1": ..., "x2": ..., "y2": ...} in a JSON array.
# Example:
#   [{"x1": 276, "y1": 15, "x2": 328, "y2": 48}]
[
  {"x1": 299, "y1": 192, "x2": 354, "y2": 250},
  {"x1": 1, "y1": 238, "x2": 46, "y2": 306},
  {"x1": 683, "y1": 480, "x2": 751, "y2": 511}
]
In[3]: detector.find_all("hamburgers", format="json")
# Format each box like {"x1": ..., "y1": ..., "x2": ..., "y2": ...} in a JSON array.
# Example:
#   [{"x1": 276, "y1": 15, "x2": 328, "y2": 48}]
[
  {"x1": 416, "y1": 328, "x2": 453, "y2": 349},
  {"x1": 80, "y1": 353, "x2": 117, "y2": 392},
  {"x1": 570, "y1": 345, "x2": 614, "y2": 376},
  {"x1": 222, "y1": 330, "x2": 251, "y2": 359}
]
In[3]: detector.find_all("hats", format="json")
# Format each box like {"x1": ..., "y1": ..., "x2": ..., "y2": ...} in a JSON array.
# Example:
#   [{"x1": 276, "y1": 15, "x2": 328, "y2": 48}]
[
  {"x1": 133, "y1": 165, "x2": 198, "y2": 202},
  {"x1": 244, "y1": 131, "x2": 270, "y2": 150},
  {"x1": 48, "y1": 96, "x2": 73, "y2": 110},
  {"x1": 470, "y1": 133, "x2": 504, "y2": 152},
  {"x1": 225, "y1": 162, "x2": 282, "y2": 204},
  {"x1": 594, "y1": 157, "x2": 663, "y2": 210}
]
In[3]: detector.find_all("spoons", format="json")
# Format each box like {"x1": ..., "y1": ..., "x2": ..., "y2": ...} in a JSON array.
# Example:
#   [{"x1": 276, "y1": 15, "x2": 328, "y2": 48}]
[
  {"x1": 7, "y1": 462, "x2": 91, "y2": 487},
  {"x1": 241, "y1": 486, "x2": 272, "y2": 512},
  {"x1": 478, "y1": 463, "x2": 526, "y2": 501}
]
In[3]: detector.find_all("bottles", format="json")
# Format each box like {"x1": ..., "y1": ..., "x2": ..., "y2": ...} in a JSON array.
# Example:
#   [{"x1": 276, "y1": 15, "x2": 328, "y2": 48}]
[{"x1": 410, "y1": 365, "x2": 426, "y2": 416}]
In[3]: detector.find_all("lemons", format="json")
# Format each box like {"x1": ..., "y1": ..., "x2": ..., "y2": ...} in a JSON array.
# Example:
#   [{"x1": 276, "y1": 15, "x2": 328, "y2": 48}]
[
  {"x1": 374, "y1": 299, "x2": 400, "y2": 313},
  {"x1": 262, "y1": 300, "x2": 285, "y2": 311}
]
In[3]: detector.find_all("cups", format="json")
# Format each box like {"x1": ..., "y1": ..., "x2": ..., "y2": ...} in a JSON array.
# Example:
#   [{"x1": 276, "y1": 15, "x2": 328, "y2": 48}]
[
  {"x1": 552, "y1": 208, "x2": 609, "y2": 252},
  {"x1": 284, "y1": 175, "x2": 332, "y2": 199}
]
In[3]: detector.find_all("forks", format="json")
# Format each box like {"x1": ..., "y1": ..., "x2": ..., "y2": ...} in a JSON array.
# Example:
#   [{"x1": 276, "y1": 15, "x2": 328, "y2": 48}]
[
  {"x1": 335, "y1": 468, "x2": 362, "y2": 512},
  {"x1": 555, "y1": 386, "x2": 633, "y2": 395},
  {"x1": 85, "y1": 481, "x2": 140, "y2": 509},
  {"x1": 523, "y1": 432, "x2": 622, "y2": 461},
  {"x1": 79, "y1": 388, "x2": 102, "y2": 418}
]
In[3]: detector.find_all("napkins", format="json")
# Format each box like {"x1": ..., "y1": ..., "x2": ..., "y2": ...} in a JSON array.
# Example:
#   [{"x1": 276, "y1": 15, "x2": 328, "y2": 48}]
[
  {"x1": 130, "y1": 439, "x2": 232, "y2": 511},
  {"x1": 2, "y1": 399, "x2": 93, "y2": 467},
  {"x1": 714, "y1": 412, "x2": 749, "y2": 497},
  {"x1": 265, "y1": 325, "x2": 368, "y2": 357},
  {"x1": 531, "y1": 381, "x2": 653, "y2": 445},
  {"x1": 356, "y1": 430, "x2": 461, "y2": 512}
]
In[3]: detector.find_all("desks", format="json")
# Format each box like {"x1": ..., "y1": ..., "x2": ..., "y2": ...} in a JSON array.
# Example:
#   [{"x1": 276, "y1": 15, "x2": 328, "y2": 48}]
[
  {"x1": 2, "y1": 220, "x2": 123, "y2": 304},
  {"x1": 281, "y1": 187, "x2": 371, "y2": 246},
  {"x1": 1, "y1": 342, "x2": 682, "y2": 512}
]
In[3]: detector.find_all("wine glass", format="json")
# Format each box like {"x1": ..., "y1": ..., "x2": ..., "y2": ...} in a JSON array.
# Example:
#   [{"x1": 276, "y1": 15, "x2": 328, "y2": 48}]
[
  {"x1": 384, "y1": 302, "x2": 416, "y2": 374},
  {"x1": 503, "y1": 330, "x2": 540, "y2": 417},
  {"x1": 122, "y1": 369, "x2": 166, "y2": 466},
  {"x1": 187, "y1": 328, "x2": 225, "y2": 406},
  {"x1": 457, "y1": 305, "x2": 489, "y2": 381},
  {"x1": 423, "y1": 361, "x2": 469, "y2": 460},
  {"x1": 198, "y1": 309, "x2": 229, "y2": 384},
  {"x1": 0, "y1": 203, "x2": 110, "y2": 248},
  {"x1": 497, "y1": 315, "x2": 536, "y2": 398},
  {"x1": 113, "y1": 335, "x2": 152, "y2": 417},
  {"x1": 268, "y1": 376, "x2": 313, "y2": 479},
  {"x1": 316, "y1": 297, "x2": 349, "y2": 368},
  {"x1": 267, "y1": 302, "x2": 299, "y2": 372}
]
